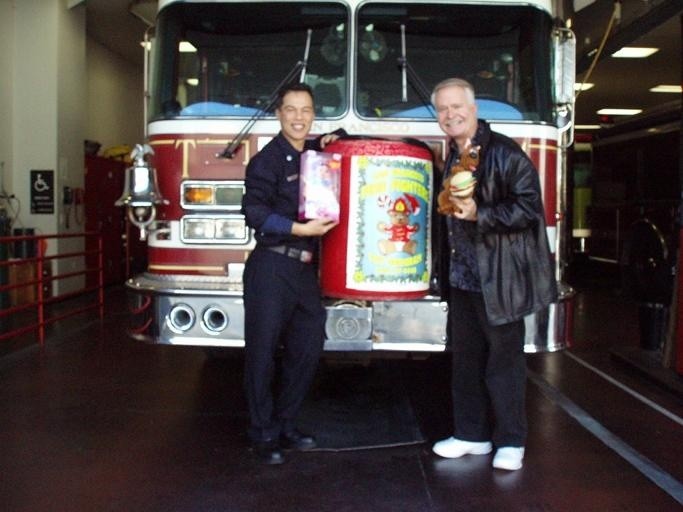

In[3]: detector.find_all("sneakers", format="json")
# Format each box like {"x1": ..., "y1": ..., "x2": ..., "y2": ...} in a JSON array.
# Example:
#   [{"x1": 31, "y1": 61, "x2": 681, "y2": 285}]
[
  {"x1": 433, "y1": 437, "x2": 493, "y2": 459},
  {"x1": 492, "y1": 445, "x2": 524, "y2": 471}
]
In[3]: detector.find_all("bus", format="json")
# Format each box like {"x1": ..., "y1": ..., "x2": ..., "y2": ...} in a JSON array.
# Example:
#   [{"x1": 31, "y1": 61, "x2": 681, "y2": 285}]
[{"x1": 112, "y1": 0, "x2": 577, "y2": 357}]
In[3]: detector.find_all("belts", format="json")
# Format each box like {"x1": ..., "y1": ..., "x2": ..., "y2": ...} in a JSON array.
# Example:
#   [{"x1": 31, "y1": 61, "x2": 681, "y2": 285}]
[{"x1": 268, "y1": 245, "x2": 315, "y2": 263}]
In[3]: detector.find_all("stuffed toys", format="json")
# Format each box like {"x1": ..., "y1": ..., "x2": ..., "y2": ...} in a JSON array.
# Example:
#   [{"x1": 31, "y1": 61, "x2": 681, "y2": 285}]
[
  {"x1": 437, "y1": 138, "x2": 481, "y2": 216},
  {"x1": 377, "y1": 197, "x2": 419, "y2": 256}
]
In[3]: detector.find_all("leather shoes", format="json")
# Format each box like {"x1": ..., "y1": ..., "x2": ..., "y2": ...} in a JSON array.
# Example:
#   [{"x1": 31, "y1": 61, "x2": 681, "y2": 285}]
[{"x1": 248, "y1": 431, "x2": 314, "y2": 465}]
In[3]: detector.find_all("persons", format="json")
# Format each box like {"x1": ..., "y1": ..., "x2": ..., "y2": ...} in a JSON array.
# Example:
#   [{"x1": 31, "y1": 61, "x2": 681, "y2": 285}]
[
  {"x1": 428, "y1": 77, "x2": 559, "y2": 473},
  {"x1": 240, "y1": 80, "x2": 347, "y2": 467}
]
